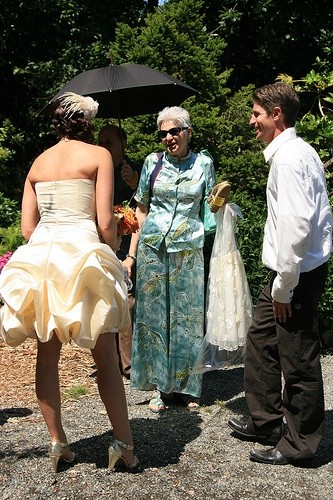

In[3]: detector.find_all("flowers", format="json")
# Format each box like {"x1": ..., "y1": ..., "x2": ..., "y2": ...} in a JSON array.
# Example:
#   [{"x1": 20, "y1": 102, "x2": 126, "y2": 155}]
[
  {"x1": 57, "y1": 91, "x2": 99, "y2": 121},
  {"x1": 97, "y1": 190, "x2": 149, "y2": 243}
]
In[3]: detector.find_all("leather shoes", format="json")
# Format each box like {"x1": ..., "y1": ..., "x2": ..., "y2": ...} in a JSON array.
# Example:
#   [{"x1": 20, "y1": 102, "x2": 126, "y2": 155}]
[
  {"x1": 227, "y1": 418, "x2": 284, "y2": 442},
  {"x1": 249, "y1": 447, "x2": 290, "y2": 465}
]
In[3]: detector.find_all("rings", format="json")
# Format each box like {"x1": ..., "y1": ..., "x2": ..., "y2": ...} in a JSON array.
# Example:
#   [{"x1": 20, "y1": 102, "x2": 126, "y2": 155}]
[{"x1": 124, "y1": 172, "x2": 126, "y2": 175}]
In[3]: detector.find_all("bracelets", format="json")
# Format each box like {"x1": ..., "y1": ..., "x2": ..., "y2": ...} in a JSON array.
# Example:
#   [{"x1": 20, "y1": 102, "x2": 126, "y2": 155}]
[{"x1": 126, "y1": 254, "x2": 137, "y2": 260}]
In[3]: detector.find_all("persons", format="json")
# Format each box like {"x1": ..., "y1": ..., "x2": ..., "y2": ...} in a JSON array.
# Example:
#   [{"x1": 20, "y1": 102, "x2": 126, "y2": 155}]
[
  {"x1": 0, "y1": 93, "x2": 143, "y2": 469},
  {"x1": 227, "y1": 82, "x2": 333, "y2": 463},
  {"x1": 95, "y1": 107, "x2": 231, "y2": 414}
]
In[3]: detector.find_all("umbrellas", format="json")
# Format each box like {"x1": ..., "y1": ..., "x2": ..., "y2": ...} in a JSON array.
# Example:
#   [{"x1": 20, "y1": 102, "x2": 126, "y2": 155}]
[{"x1": 39, "y1": 53, "x2": 202, "y2": 164}]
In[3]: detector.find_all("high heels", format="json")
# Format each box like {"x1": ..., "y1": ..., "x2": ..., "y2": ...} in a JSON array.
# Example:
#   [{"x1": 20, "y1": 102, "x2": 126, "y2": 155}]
[
  {"x1": 108, "y1": 439, "x2": 141, "y2": 473},
  {"x1": 48, "y1": 442, "x2": 75, "y2": 472}
]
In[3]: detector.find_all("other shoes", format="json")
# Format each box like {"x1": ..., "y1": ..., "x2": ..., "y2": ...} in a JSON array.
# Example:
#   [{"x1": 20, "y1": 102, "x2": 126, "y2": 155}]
[
  {"x1": 207, "y1": 181, "x2": 231, "y2": 213},
  {"x1": 149, "y1": 392, "x2": 174, "y2": 413},
  {"x1": 178, "y1": 393, "x2": 199, "y2": 408}
]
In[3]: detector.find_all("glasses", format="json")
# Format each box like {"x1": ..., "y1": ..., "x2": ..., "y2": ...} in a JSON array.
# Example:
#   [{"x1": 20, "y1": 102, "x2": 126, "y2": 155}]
[{"x1": 157, "y1": 127, "x2": 188, "y2": 138}]
